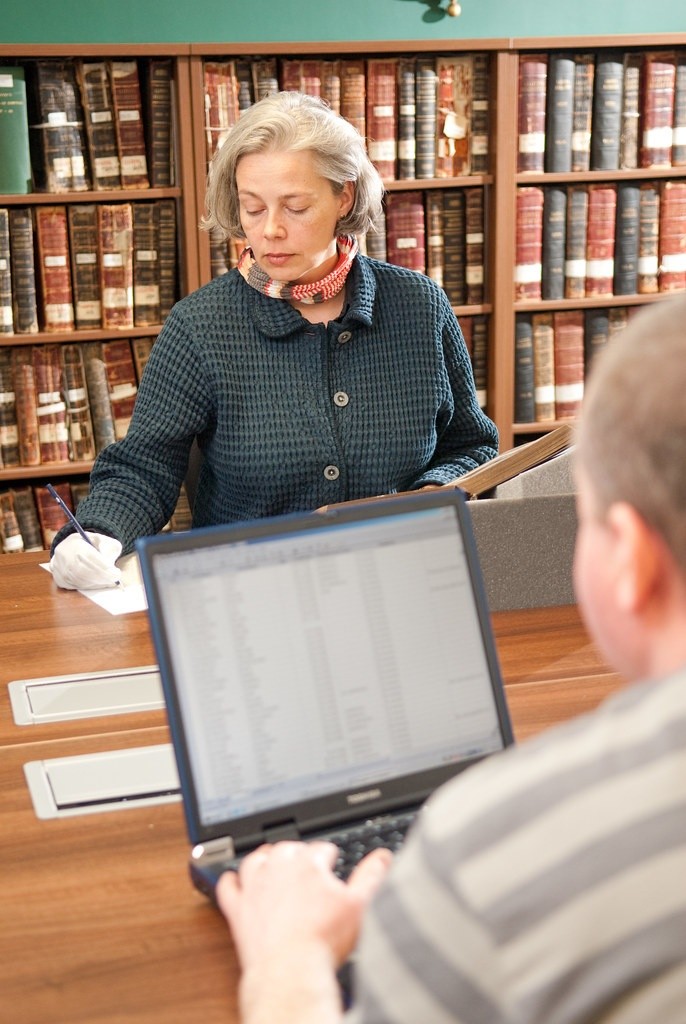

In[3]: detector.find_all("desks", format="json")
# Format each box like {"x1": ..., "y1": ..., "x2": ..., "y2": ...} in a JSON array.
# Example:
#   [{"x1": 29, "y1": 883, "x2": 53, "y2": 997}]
[{"x1": 0, "y1": 547, "x2": 628, "y2": 1024}]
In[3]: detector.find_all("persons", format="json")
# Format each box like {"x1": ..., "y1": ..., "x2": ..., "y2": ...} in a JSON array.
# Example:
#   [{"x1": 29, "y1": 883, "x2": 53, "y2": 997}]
[
  {"x1": 216, "y1": 285, "x2": 686, "y2": 1024},
  {"x1": 50, "y1": 90, "x2": 500, "y2": 590}
]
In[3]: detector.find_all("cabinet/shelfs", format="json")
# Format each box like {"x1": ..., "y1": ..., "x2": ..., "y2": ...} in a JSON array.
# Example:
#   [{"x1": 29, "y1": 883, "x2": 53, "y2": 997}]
[{"x1": 0, "y1": 34, "x2": 686, "y2": 561}]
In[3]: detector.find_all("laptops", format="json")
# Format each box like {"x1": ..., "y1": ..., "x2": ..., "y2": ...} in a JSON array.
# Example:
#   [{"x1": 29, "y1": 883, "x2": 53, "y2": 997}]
[{"x1": 136, "y1": 489, "x2": 517, "y2": 901}]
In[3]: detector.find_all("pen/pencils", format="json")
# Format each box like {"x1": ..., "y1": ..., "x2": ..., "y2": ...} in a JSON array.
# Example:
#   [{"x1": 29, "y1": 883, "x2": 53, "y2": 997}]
[{"x1": 47, "y1": 483, "x2": 120, "y2": 586}]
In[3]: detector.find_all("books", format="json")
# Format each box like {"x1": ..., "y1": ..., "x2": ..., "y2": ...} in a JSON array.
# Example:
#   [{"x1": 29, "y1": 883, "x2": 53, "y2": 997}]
[
  {"x1": 315, "y1": 424, "x2": 577, "y2": 512},
  {"x1": 0, "y1": 55, "x2": 686, "y2": 549}
]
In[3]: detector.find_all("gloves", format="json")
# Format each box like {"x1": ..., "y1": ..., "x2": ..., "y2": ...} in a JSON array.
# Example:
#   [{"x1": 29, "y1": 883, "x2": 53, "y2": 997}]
[{"x1": 51, "y1": 531, "x2": 123, "y2": 590}]
